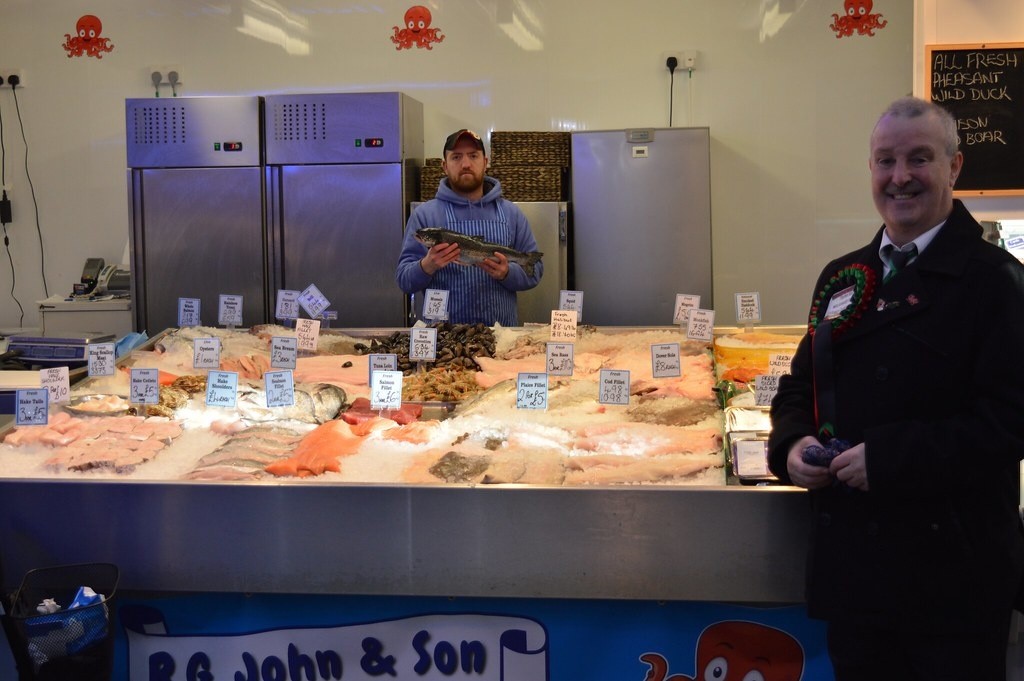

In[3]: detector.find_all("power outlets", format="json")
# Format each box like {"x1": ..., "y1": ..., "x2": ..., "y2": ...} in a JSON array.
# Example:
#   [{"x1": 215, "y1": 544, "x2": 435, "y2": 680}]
[
  {"x1": 0, "y1": 68, "x2": 5, "y2": 89},
  {"x1": 662, "y1": 50, "x2": 698, "y2": 71},
  {"x1": 149, "y1": 65, "x2": 185, "y2": 85},
  {"x1": 6, "y1": 68, "x2": 22, "y2": 89}
]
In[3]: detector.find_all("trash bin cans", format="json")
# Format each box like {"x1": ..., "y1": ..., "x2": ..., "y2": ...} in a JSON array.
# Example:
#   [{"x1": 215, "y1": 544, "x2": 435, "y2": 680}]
[{"x1": 10, "y1": 562, "x2": 120, "y2": 681}]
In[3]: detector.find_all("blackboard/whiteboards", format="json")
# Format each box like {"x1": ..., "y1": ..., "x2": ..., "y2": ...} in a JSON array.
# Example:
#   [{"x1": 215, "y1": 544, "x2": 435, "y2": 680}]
[{"x1": 924, "y1": 43, "x2": 1024, "y2": 197}]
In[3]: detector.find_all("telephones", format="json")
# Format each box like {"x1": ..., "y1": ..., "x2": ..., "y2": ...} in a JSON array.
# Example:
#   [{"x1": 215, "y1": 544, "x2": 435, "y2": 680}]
[{"x1": 97, "y1": 263, "x2": 130, "y2": 295}]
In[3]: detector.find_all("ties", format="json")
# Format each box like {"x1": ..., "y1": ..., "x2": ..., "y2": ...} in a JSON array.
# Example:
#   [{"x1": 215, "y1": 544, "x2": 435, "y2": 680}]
[{"x1": 883, "y1": 247, "x2": 919, "y2": 286}]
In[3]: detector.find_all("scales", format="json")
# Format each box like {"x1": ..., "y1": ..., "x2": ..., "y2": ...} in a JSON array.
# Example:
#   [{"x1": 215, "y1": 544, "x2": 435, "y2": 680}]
[{"x1": 4, "y1": 329, "x2": 118, "y2": 365}]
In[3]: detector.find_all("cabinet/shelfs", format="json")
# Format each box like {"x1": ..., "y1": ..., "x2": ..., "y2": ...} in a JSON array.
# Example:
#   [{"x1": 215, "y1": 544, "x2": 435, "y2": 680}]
[{"x1": 39, "y1": 298, "x2": 131, "y2": 338}]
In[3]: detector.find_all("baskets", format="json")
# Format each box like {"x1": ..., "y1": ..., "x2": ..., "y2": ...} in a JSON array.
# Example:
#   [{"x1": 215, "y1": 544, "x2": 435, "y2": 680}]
[{"x1": 11, "y1": 562, "x2": 118, "y2": 680}]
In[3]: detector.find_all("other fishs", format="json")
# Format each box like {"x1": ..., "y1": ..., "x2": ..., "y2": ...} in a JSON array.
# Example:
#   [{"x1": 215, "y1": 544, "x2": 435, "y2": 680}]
[
  {"x1": 413, "y1": 226, "x2": 546, "y2": 279},
  {"x1": 234, "y1": 381, "x2": 348, "y2": 427}
]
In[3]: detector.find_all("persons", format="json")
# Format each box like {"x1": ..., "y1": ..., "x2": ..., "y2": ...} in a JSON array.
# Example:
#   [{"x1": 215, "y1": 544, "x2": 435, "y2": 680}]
[
  {"x1": 763, "y1": 94, "x2": 1024, "y2": 681},
  {"x1": 396, "y1": 128, "x2": 544, "y2": 327}
]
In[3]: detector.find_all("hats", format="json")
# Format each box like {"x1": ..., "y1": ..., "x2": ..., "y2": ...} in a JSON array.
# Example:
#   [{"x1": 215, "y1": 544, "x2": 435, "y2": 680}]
[{"x1": 445, "y1": 129, "x2": 483, "y2": 150}]
